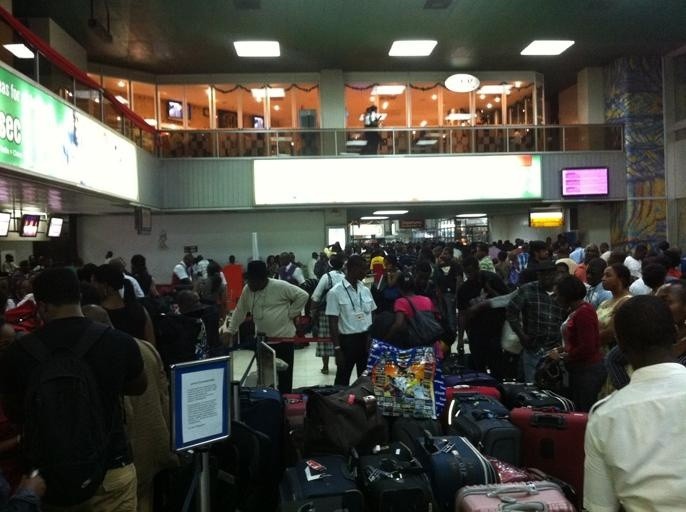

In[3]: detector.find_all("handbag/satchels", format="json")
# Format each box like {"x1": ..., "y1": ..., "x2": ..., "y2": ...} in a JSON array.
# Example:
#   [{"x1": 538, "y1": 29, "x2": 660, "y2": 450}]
[
  {"x1": 368, "y1": 339, "x2": 446, "y2": 419},
  {"x1": 389, "y1": 311, "x2": 445, "y2": 348},
  {"x1": 371, "y1": 121, "x2": 378, "y2": 127},
  {"x1": 536, "y1": 352, "x2": 572, "y2": 398}
]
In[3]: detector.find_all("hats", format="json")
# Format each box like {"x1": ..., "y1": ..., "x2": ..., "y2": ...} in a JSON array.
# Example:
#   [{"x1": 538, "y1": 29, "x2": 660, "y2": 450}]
[
  {"x1": 329, "y1": 255, "x2": 344, "y2": 269},
  {"x1": 242, "y1": 260, "x2": 271, "y2": 280},
  {"x1": 178, "y1": 290, "x2": 210, "y2": 314},
  {"x1": 533, "y1": 258, "x2": 556, "y2": 271}
]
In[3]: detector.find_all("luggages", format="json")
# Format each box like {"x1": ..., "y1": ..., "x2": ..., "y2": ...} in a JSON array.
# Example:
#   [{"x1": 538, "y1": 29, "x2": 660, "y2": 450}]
[
  {"x1": 278, "y1": 450, "x2": 362, "y2": 512},
  {"x1": 242, "y1": 385, "x2": 284, "y2": 416},
  {"x1": 281, "y1": 390, "x2": 307, "y2": 412},
  {"x1": 449, "y1": 393, "x2": 523, "y2": 470},
  {"x1": 511, "y1": 407, "x2": 589, "y2": 510},
  {"x1": 356, "y1": 440, "x2": 433, "y2": 512},
  {"x1": 292, "y1": 383, "x2": 378, "y2": 452},
  {"x1": 503, "y1": 378, "x2": 579, "y2": 413},
  {"x1": 419, "y1": 429, "x2": 501, "y2": 511},
  {"x1": 456, "y1": 480, "x2": 575, "y2": 512},
  {"x1": 447, "y1": 385, "x2": 501, "y2": 402},
  {"x1": 209, "y1": 381, "x2": 277, "y2": 511},
  {"x1": 445, "y1": 369, "x2": 499, "y2": 388}
]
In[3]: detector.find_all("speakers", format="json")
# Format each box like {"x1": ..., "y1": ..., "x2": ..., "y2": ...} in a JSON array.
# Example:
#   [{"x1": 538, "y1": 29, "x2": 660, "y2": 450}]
[{"x1": 569, "y1": 207, "x2": 578, "y2": 230}]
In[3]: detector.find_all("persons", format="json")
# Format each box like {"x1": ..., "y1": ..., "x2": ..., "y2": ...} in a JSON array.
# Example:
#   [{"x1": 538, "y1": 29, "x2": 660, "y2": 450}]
[
  {"x1": 361, "y1": 106, "x2": 384, "y2": 155},
  {"x1": 476, "y1": 108, "x2": 487, "y2": 124},
  {"x1": 0, "y1": 237, "x2": 686, "y2": 512},
  {"x1": 22, "y1": 219, "x2": 38, "y2": 236}
]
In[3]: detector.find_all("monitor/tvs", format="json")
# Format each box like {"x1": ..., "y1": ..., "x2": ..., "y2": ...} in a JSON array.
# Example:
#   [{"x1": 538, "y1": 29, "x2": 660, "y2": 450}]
[
  {"x1": 47, "y1": 217, "x2": 64, "y2": 237},
  {"x1": 167, "y1": 100, "x2": 191, "y2": 119},
  {"x1": 560, "y1": 166, "x2": 609, "y2": 198},
  {"x1": 0, "y1": 212, "x2": 11, "y2": 237},
  {"x1": 136, "y1": 206, "x2": 153, "y2": 235},
  {"x1": 20, "y1": 214, "x2": 40, "y2": 236},
  {"x1": 252, "y1": 115, "x2": 265, "y2": 129}
]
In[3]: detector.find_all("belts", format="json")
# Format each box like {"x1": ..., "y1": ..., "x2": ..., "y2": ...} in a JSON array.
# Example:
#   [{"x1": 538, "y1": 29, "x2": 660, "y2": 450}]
[{"x1": 104, "y1": 454, "x2": 134, "y2": 473}]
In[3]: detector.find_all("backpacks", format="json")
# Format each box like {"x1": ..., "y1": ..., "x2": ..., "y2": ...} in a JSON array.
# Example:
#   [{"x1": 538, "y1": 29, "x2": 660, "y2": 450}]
[{"x1": 17, "y1": 320, "x2": 123, "y2": 504}]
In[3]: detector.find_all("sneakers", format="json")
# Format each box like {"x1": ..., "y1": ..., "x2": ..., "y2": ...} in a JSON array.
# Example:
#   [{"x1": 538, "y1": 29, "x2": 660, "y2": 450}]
[{"x1": 320, "y1": 367, "x2": 328, "y2": 374}]
